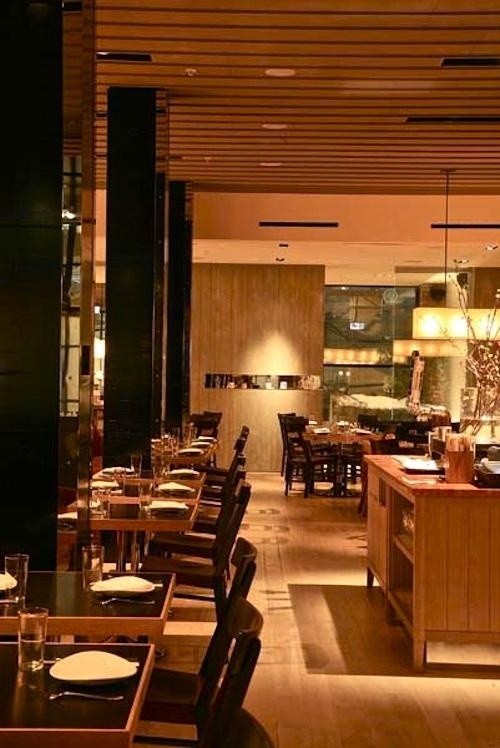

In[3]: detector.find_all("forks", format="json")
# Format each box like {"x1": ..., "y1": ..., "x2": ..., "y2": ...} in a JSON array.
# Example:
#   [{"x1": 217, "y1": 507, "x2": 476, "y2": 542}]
[
  {"x1": 29, "y1": 685, "x2": 125, "y2": 704},
  {"x1": 90, "y1": 596, "x2": 156, "y2": 607}
]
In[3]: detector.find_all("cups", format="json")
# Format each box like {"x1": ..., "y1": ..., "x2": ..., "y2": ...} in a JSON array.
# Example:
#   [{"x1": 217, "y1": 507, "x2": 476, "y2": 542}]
[
  {"x1": 279, "y1": 380, "x2": 288, "y2": 390},
  {"x1": 87, "y1": 452, "x2": 142, "y2": 518},
  {"x1": 15, "y1": 607, "x2": 49, "y2": 674},
  {"x1": 309, "y1": 412, "x2": 360, "y2": 443},
  {"x1": 81, "y1": 545, "x2": 105, "y2": 593},
  {"x1": 4, "y1": 553, "x2": 29, "y2": 606},
  {"x1": 241, "y1": 382, "x2": 248, "y2": 389},
  {"x1": 152, "y1": 421, "x2": 197, "y2": 463}
]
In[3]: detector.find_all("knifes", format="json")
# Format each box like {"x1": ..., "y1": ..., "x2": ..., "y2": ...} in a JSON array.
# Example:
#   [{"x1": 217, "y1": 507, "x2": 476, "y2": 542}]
[{"x1": 43, "y1": 657, "x2": 140, "y2": 667}]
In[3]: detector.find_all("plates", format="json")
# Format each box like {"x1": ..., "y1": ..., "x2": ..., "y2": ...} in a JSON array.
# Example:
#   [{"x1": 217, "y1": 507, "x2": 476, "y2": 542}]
[
  {"x1": 87, "y1": 575, "x2": 156, "y2": 597},
  {"x1": 177, "y1": 436, "x2": 216, "y2": 455},
  {"x1": 474, "y1": 467, "x2": 500, "y2": 484},
  {"x1": 147, "y1": 469, "x2": 200, "y2": 515},
  {"x1": 40, "y1": 650, "x2": 143, "y2": 688}
]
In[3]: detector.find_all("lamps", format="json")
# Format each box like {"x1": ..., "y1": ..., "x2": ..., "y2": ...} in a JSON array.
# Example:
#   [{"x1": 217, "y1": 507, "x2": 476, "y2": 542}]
[{"x1": 412, "y1": 170, "x2": 500, "y2": 340}]
